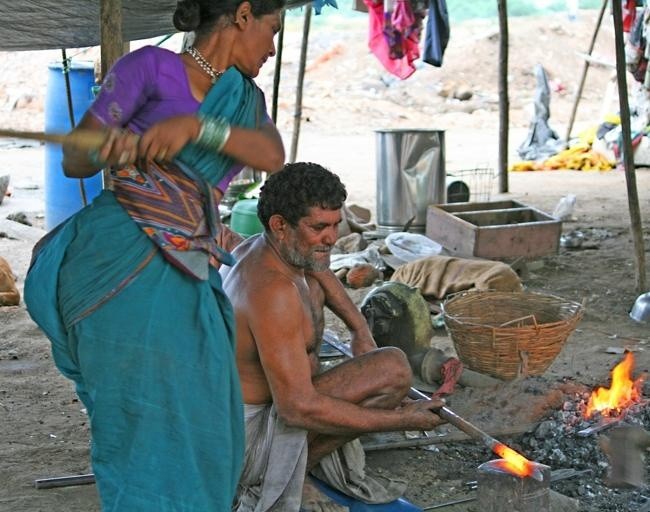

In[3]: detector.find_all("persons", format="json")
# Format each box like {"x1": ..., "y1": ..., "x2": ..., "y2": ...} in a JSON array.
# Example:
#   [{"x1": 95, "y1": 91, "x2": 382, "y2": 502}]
[
  {"x1": 213, "y1": 161, "x2": 451, "y2": 512},
  {"x1": 20, "y1": 0, "x2": 286, "y2": 512}
]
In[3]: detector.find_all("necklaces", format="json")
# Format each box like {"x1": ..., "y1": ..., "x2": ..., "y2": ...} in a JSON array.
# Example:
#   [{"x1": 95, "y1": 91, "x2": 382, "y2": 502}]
[{"x1": 180, "y1": 41, "x2": 237, "y2": 85}]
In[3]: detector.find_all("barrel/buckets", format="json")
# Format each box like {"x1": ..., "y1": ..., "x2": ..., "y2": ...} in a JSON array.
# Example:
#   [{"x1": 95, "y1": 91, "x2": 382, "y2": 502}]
[
  {"x1": 231, "y1": 198, "x2": 264, "y2": 241},
  {"x1": 43, "y1": 61, "x2": 104, "y2": 235}
]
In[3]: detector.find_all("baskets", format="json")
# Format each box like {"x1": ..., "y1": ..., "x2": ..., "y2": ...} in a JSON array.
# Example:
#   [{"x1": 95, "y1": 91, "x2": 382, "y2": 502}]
[{"x1": 440, "y1": 287, "x2": 589, "y2": 380}]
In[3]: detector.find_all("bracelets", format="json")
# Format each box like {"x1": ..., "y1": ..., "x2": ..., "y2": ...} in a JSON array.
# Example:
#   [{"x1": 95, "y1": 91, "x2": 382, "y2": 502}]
[{"x1": 192, "y1": 113, "x2": 231, "y2": 155}]
